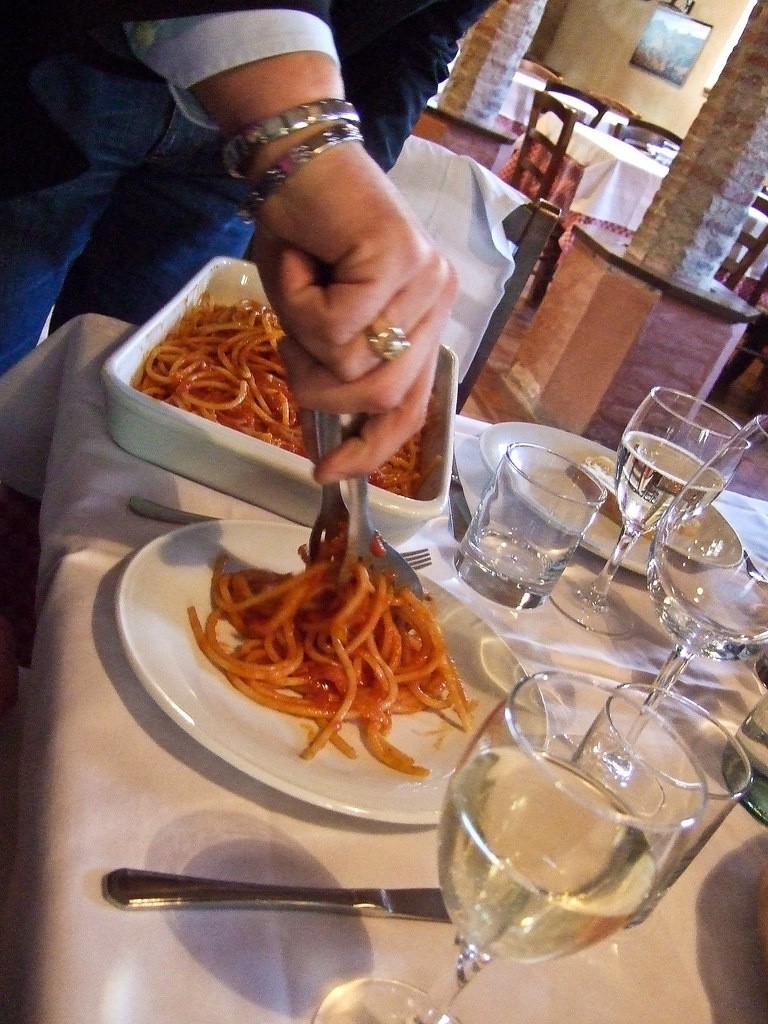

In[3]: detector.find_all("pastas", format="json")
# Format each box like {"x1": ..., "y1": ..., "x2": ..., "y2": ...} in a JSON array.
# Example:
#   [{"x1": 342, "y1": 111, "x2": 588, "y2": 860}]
[
  {"x1": 189, "y1": 519, "x2": 477, "y2": 777},
  {"x1": 137, "y1": 291, "x2": 424, "y2": 501}
]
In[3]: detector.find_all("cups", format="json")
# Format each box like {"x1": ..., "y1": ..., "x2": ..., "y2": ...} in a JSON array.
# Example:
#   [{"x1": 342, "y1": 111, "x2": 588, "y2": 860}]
[{"x1": 450, "y1": 442, "x2": 606, "y2": 612}]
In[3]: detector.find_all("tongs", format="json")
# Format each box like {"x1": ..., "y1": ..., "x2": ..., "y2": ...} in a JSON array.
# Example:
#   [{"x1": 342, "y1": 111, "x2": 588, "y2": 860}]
[{"x1": 305, "y1": 409, "x2": 423, "y2": 633}]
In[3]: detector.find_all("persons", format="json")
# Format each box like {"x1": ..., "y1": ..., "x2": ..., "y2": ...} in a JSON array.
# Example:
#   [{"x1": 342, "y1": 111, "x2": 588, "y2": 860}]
[{"x1": 0, "y1": 0, "x2": 499, "y2": 483}]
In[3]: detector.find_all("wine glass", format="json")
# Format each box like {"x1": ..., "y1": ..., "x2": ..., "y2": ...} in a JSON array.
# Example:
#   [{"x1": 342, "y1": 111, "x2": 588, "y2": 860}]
[
  {"x1": 311, "y1": 671, "x2": 755, "y2": 1024},
  {"x1": 545, "y1": 385, "x2": 752, "y2": 636},
  {"x1": 546, "y1": 414, "x2": 768, "y2": 816}
]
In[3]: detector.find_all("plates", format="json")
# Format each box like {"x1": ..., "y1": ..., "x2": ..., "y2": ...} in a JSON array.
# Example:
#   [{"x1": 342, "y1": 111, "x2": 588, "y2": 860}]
[
  {"x1": 112, "y1": 519, "x2": 550, "y2": 827},
  {"x1": 100, "y1": 255, "x2": 460, "y2": 547},
  {"x1": 476, "y1": 418, "x2": 743, "y2": 576}
]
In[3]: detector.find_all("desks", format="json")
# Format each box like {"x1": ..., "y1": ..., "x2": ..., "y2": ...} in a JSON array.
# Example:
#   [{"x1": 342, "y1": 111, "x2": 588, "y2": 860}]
[
  {"x1": 427, "y1": 51, "x2": 679, "y2": 152},
  {"x1": 495, "y1": 112, "x2": 669, "y2": 252}
]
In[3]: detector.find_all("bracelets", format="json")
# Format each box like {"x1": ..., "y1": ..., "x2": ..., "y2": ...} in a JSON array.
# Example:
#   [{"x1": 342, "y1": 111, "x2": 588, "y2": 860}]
[
  {"x1": 244, "y1": 125, "x2": 363, "y2": 238},
  {"x1": 222, "y1": 99, "x2": 360, "y2": 178}
]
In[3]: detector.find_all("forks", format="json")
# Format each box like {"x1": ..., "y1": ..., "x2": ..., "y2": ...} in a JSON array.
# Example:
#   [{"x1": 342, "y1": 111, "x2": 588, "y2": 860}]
[
  {"x1": 391, "y1": 547, "x2": 431, "y2": 572},
  {"x1": 127, "y1": 493, "x2": 433, "y2": 573}
]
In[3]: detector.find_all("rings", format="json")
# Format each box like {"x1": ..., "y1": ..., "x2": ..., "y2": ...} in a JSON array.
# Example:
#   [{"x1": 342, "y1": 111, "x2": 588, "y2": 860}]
[{"x1": 367, "y1": 324, "x2": 408, "y2": 362}]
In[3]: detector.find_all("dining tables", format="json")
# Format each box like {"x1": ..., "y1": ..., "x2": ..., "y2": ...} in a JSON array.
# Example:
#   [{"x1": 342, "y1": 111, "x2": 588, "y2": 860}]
[{"x1": 0, "y1": 313, "x2": 768, "y2": 1024}]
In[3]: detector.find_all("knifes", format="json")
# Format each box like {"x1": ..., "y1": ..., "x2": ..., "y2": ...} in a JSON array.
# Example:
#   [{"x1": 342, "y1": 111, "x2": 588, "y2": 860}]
[{"x1": 101, "y1": 867, "x2": 451, "y2": 925}]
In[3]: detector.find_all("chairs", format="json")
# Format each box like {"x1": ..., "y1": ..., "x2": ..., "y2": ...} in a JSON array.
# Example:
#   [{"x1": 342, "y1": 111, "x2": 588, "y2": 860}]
[
  {"x1": 614, "y1": 122, "x2": 666, "y2": 159},
  {"x1": 720, "y1": 196, "x2": 768, "y2": 290},
  {"x1": 541, "y1": 79, "x2": 610, "y2": 130},
  {"x1": 510, "y1": 89, "x2": 578, "y2": 309},
  {"x1": 386, "y1": 136, "x2": 563, "y2": 415},
  {"x1": 589, "y1": 90, "x2": 642, "y2": 121},
  {"x1": 519, "y1": 58, "x2": 561, "y2": 83}
]
